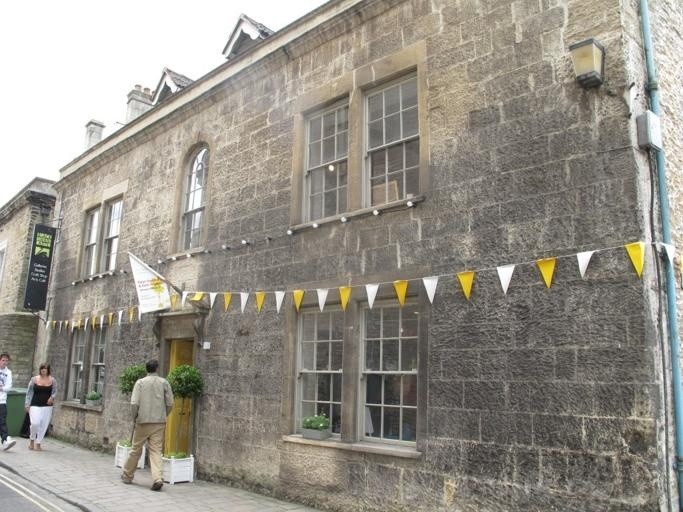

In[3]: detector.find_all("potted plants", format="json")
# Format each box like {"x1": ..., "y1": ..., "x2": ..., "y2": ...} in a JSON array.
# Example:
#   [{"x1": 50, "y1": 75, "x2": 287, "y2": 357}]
[
  {"x1": 83, "y1": 390, "x2": 102, "y2": 406},
  {"x1": 115, "y1": 363, "x2": 146, "y2": 469},
  {"x1": 302, "y1": 412, "x2": 332, "y2": 440},
  {"x1": 163, "y1": 364, "x2": 202, "y2": 485}
]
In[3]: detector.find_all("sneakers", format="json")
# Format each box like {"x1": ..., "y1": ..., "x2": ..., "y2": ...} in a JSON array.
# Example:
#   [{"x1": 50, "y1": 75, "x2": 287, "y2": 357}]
[
  {"x1": 153, "y1": 482, "x2": 162, "y2": 490},
  {"x1": 3, "y1": 436, "x2": 16, "y2": 450}
]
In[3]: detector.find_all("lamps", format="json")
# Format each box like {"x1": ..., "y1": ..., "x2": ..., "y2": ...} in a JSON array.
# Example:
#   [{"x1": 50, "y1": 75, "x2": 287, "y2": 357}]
[{"x1": 568, "y1": 38, "x2": 607, "y2": 90}]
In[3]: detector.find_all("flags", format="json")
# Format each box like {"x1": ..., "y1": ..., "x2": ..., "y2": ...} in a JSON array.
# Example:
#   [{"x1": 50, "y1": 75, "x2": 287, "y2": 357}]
[{"x1": 129, "y1": 251, "x2": 171, "y2": 313}]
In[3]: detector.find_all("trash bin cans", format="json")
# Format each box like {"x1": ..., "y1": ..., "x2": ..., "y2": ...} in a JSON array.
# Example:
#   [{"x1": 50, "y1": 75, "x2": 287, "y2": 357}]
[{"x1": 5, "y1": 388, "x2": 27, "y2": 437}]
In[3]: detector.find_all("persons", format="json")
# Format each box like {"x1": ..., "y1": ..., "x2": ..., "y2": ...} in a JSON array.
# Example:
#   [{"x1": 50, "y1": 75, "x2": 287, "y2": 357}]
[
  {"x1": 25, "y1": 363, "x2": 57, "y2": 451},
  {"x1": 0, "y1": 352, "x2": 16, "y2": 451},
  {"x1": 121, "y1": 360, "x2": 174, "y2": 490}
]
